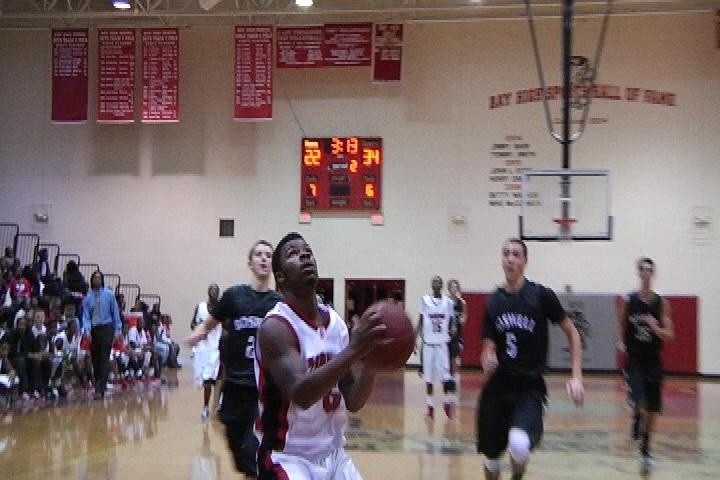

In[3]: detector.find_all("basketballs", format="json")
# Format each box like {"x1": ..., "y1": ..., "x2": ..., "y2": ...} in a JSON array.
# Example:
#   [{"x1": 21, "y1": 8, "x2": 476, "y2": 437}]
[{"x1": 357, "y1": 300, "x2": 413, "y2": 371}]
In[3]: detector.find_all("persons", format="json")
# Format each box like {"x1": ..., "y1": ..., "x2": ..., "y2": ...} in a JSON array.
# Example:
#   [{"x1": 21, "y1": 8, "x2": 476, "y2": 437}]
[
  {"x1": 617, "y1": 257, "x2": 673, "y2": 462},
  {"x1": 475, "y1": 237, "x2": 586, "y2": 480},
  {"x1": 185, "y1": 240, "x2": 286, "y2": 480},
  {"x1": 190, "y1": 283, "x2": 225, "y2": 419},
  {"x1": 413, "y1": 278, "x2": 467, "y2": 418},
  {"x1": 0, "y1": 247, "x2": 181, "y2": 404},
  {"x1": 252, "y1": 233, "x2": 404, "y2": 480}
]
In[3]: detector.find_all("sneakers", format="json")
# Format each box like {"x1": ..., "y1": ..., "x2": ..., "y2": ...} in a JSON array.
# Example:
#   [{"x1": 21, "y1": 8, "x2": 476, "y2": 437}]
[
  {"x1": 424, "y1": 414, "x2": 457, "y2": 426},
  {"x1": 202, "y1": 408, "x2": 209, "y2": 419},
  {"x1": 631, "y1": 414, "x2": 656, "y2": 466}
]
